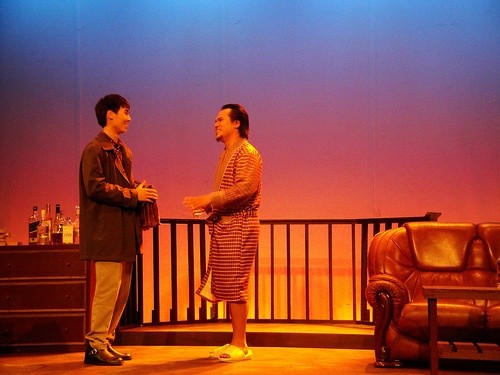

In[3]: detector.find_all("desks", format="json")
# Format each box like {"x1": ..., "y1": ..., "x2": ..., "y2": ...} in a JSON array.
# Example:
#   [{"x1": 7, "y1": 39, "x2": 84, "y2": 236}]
[{"x1": 423, "y1": 286, "x2": 500, "y2": 375}]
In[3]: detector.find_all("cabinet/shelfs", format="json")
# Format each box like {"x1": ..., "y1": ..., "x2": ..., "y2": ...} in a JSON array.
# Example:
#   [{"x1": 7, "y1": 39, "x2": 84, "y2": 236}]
[{"x1": 0, "y1": 245, "x2": 92, "y2": 356}]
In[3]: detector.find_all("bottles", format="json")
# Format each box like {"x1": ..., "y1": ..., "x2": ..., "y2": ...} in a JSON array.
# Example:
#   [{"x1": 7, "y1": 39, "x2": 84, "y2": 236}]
[{"x1": 28, "y1": 203, "x2": 80, "y2": 245}]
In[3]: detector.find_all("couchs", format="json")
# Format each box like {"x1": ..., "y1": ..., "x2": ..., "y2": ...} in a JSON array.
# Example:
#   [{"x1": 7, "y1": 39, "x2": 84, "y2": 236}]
[{"x1": 365, "y1": 222, "x2": 500, "y2": 367}]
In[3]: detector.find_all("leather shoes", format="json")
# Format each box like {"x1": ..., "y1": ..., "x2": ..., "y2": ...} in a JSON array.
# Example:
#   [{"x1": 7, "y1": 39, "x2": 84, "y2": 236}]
[
  {"x1": 108, "y1": 346, "x2": 132, "y2": 360},
  {"x1": 83, "y1": 347, "x2": 122, "y2": 365}
]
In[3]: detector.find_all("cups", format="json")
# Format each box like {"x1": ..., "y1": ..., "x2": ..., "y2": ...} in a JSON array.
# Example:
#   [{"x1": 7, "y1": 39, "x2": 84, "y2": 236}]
[{"x1": 192, "y1": 207, "x2": 204, "y2": 215}]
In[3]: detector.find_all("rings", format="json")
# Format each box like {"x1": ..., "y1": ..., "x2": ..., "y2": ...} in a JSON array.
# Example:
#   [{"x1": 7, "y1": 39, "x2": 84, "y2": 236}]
[{"x1": 192, "y1": 206, "x2": 193, "y2": 210}]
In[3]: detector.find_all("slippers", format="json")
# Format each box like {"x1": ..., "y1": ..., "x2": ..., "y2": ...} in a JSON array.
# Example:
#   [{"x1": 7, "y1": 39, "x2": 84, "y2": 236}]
[
  {"x1": 218, "y1": 345, "x2": 253, "y2": 362},
  {"x1": 209, "y1": 344, "x2": 231, "y2": 358}
]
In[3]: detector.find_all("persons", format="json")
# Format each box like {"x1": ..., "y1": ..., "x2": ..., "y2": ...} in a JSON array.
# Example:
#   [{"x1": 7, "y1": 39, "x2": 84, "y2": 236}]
[
  {"x1": 183, "y1": 104, "x2": 262, "y2": 362},
  {"x1": 80, "y1": 94, "x2": 158, "y2": 366}
]
